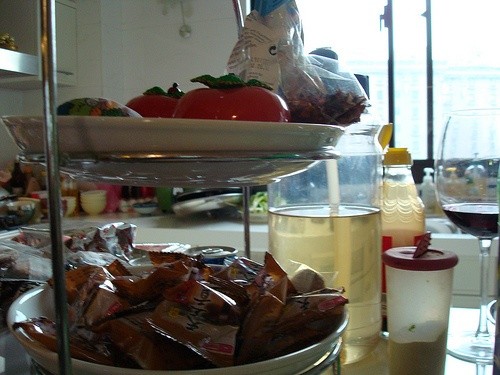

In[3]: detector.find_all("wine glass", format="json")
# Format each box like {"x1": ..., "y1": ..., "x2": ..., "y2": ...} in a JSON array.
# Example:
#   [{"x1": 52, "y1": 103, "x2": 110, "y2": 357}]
[{"x1": 435, "y1": 111, "x2": 500, "y2": 364}]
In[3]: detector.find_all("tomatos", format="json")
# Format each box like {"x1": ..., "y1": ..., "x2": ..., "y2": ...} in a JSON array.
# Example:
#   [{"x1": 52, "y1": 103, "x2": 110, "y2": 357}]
[{"x1": 121, "y1": 73, "x2": 290, "y2": 124}]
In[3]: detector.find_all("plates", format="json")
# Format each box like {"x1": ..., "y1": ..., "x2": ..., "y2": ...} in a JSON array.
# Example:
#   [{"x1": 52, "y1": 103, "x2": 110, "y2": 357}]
[
  {"x1": 1, "y1": 114, "x2": 344, "y2": 182},
  {"x1": 7, "y1": 284, "x2": 351, "y2": 375}
]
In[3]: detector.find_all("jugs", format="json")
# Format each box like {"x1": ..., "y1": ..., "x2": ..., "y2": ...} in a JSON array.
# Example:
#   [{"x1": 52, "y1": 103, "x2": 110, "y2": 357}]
[{"x1": 262, "y1": 123, "x2": 382, "y2": 364}]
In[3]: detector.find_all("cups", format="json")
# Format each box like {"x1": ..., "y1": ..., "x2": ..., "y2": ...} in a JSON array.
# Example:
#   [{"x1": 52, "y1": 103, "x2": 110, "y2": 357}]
[{"x1": 383, "y1": 244, "x2": 456, "y2": 375}]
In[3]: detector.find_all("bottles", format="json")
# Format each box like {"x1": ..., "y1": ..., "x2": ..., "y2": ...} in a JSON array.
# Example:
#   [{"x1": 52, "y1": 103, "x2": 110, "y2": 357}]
[{"x1": 383, "y1": 124, "x2": 424, "y2": 333}]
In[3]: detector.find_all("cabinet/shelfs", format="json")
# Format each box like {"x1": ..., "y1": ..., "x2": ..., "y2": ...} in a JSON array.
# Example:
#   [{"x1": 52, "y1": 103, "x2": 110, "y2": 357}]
[{"x1": 0, "y1": 0, "x2": 76, "y2": 90}]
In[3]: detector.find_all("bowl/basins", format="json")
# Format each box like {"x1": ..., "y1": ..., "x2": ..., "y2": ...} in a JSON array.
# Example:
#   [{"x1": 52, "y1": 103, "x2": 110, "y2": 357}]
[
  {"x1": 486, "y1": 299, "x2": 497, "y2": 324},
  {"x1": 0, "y1": 183, "x2": 158, "y2": 227}
]
